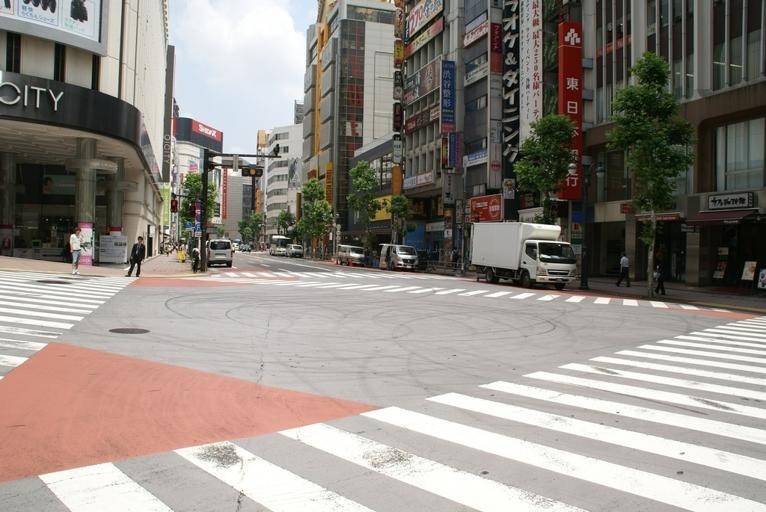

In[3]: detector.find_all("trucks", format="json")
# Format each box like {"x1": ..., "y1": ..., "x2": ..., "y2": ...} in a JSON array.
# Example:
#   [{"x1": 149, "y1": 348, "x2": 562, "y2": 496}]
[{"x1": 469, "y1": 221, "x2": 576, "y2": 290}]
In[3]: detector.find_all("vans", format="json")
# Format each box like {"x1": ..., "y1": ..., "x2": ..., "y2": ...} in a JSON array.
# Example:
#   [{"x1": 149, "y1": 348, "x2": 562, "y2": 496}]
[
  {"x1": 338, "y1": 244, "x2": 366, "y2": 266},
  {"x1": 207, "y1": 239, "x2": 232, "y2": 267},
  {"x1": 286, "y1": 244, "x2": 303, "y2": 258},
  {"x1": 379, "y1": 244, "x2": 419, "y2": 271}
]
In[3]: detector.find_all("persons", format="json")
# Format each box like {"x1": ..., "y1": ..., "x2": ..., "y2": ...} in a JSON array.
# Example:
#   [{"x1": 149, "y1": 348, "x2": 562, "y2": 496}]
[
  {"x1": 158, "y1": 235, "x2": 188, "y2": 263},
  {"x1": 655, "y1": 251, "x2": 665, "y2": 295},
  {"x1": 449, "y1": 246, "x2": 461, "y2": 272},
  {"x1": 125, "y1": 237, "x2": 145, "y2": 278},
  {"x1": 615, "y1": 252, "x2": 633, "y2": 289},
  {"x1": 67, "y1": 226, "x2": 85, "y2": 276}
]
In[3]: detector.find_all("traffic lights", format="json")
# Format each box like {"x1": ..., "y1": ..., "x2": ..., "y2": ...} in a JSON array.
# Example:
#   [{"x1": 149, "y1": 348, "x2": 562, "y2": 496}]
[
  {"x1": 242, "y1": 168, "x2": 263, "y2": 177},
  {"x1": 171, "y1": 199, "x2": 177, "y2": 212},
  {"x1": 190, "y1": 205, "x2": 196, "y2": 218}
]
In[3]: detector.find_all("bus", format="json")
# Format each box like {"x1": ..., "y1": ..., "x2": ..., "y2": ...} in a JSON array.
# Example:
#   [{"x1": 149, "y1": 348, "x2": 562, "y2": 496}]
[{"x1": 269, "y1": 235, "x2": 291, "y2": 256}]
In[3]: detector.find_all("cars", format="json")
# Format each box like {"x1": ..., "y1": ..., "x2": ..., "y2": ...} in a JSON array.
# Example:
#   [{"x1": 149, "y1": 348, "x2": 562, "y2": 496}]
[{"x1": 242, "y1": 245, "x2": 251, "y2": 252}]
